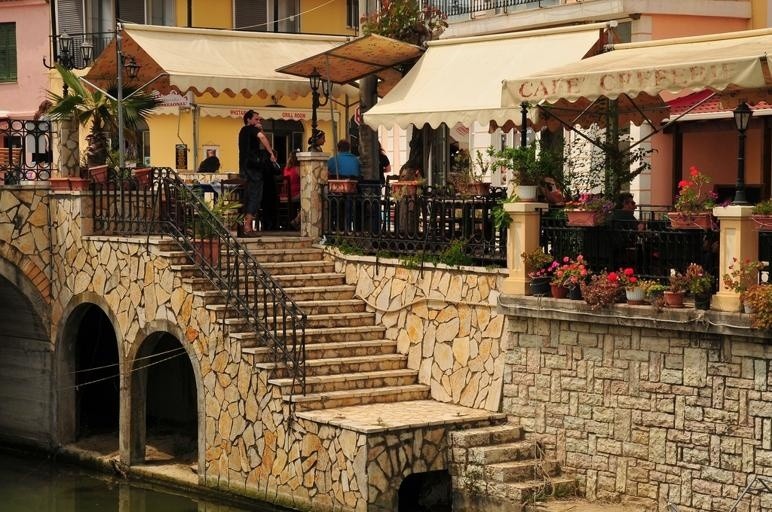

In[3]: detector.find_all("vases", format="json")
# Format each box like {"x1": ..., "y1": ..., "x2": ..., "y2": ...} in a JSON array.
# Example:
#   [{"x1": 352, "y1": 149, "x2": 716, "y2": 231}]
[
  {"x1": 89, "y1": 164, "x2": 108, "y2": 183},
  {"x1": 328, "y1": 179, "x2": 358, "y2": 193},
  {"x1": 68, "y1": 178, "x2": 92, "y2": 190},
  {"x1": 667, "y1": 211, "x2": 714, "y2": 230},
  {"x1": 48, "y1": 177, "x2": 72, "y2": 191},
  {"x1": 383, "y1": 30, "x2": 419, "y2": 45},
  {"x1": 563, "y1": 208, "x2": 605, "y2": 226}
]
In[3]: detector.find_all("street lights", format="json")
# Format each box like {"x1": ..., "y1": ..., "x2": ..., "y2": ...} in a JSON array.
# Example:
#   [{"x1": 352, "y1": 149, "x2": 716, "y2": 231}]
[
  {"x1": 305, "y1": 65, "x2": 335, "y2": 154},
  {"x1": 114, "y1": 21, "x2": 144, "y2": 170},
  {"x1": 41, "y1": 27, "x2": 95, "y2": 99},
  {"x1": 727, "y1": 98, "x2": 754, "y2": 207}
]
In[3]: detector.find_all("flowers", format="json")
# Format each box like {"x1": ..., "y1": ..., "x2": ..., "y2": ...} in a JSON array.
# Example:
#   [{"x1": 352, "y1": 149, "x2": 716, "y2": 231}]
[
  {"x1": 359, "y1": 0, "x2": 448, "y2": 48},
  {"x1": 520, "y1": 246, "x2": 763, "y2": 314},
  {"x1": 562, "y1": 193, "x2": 616, "y2": 214},
  {"x1": 674, "y1": 165, "x2": 719, "y2": 225}
]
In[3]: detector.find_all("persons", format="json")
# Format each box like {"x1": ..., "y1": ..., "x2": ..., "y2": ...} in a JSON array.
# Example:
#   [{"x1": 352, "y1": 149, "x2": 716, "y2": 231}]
[
  {"x1": 237, "y1": 110, "x2": 301, "y2": 238},
  {"x1": 191, "y1": 156, "x2": 220, "y2": 205},
  {"x1": 308, "y1": 129, "x2": 326, "y2": 152},
  {"x1": 27, "y1": 99, "x2": 53, "y2": 182},
  {"x1": 327, "y1": 139, "x2": 360, "y2": 179},
  {"x1": 604, "y1": 192, "x2": 645, "y2": 232},
  {"x1": 378, "y1": 141, "x2": 391, "y2": 187}
]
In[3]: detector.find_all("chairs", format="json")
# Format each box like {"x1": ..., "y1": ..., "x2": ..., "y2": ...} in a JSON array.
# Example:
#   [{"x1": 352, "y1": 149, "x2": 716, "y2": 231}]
[{"x1": 221, "y1": 172, "x2": 300, "y2": 231}]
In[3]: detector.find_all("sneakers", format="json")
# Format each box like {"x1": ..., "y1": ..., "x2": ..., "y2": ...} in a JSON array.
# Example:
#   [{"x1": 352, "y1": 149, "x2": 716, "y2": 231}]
[{"x1": 239, "y1": 230, "x2": 262, "y2": 237}]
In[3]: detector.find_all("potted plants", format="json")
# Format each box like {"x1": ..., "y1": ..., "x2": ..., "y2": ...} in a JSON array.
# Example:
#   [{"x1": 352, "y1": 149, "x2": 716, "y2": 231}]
[
  {"x1": 106, "y1": 149, "x2": 130, "y2": 191},
  {"x1": 133, "y1": 155, "x2": 151, "y2": 191},
  {"x1": 178, "y1": 185, "x2": 243, "y2": 267},
  {"x1": 486, "y1": 140, "x2": 574, "y2": 201},
  {"x1": 390, "y1": 178, "x2": 427, "y2": 204},
  {"x1": 470, "y1": 150, "x2": 492, "y2": 196},
  {"x1": 750, "y1": 199, "x2": 772, "y2": 232}
]
